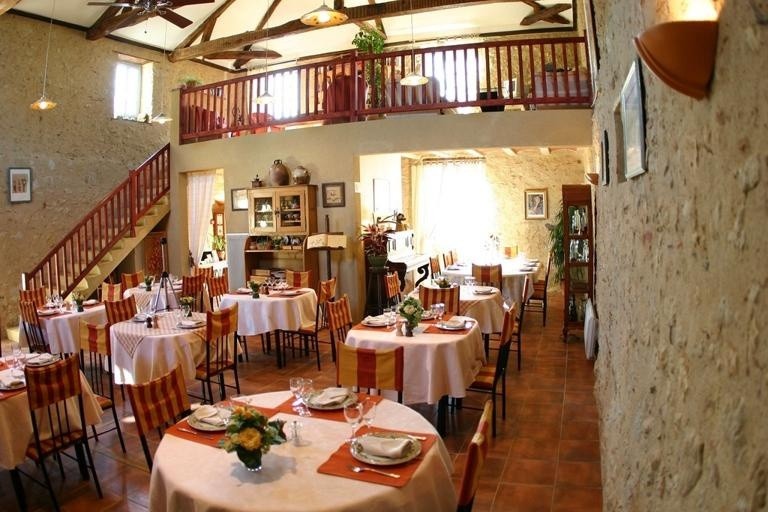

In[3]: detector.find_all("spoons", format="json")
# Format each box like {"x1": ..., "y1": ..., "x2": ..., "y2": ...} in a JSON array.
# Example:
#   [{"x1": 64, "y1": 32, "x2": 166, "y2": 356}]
[{"x1": 347, "y1": 465, "x2": 400, "y2": 479}]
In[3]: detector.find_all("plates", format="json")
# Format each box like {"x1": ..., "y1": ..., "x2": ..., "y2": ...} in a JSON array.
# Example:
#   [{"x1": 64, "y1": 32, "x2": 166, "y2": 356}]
[
  {"x1": 519, "y1": 259, "x2": 539, "y2": 272},
  {"x1": 474, "y1": 286, "x2": 492, "y2": 292},
  {"x1": 177, "y1": 320, "x2": 207, "y2": 328},
  {"x1": 0, "y1": 368, "x2": 27, "y2": 390},
  {"x1": 361, "y1": 313, "x2": 396, "y2": 326},
  {"x1": 26, "y1": 355, "x2": 61, "y2": 366},
  {"x1": 186, "y1": 388, "x2": 422, "y2": 465}
]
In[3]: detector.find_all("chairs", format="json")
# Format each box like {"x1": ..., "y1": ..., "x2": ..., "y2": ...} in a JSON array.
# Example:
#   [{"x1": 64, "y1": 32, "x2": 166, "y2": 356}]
[
  {"x1": 324, "y1": 76, "x2": 369, "y2": 124},
  {"x1": 248, "y1": 112, "x2": 276, "y2": 135}
]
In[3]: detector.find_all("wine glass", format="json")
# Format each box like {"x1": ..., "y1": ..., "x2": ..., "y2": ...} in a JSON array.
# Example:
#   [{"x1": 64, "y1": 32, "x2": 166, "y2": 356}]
[
  {"x1": 216, "y1": 378, "x2": 375, "y2": 446},
  {"x1": 172, "y1": 276, "x2": 178, "y2": 284},
  {"x1": 174, "y1": 309, "x2": 184, "y2": 326},
  {"x1": 150, "y1": 276, "x2": 155, "y2": 287},
  {"x1": 12, "y1": 344, "x2": 21, "y2": 368},
  {"x1": 383, "y1": 272, "x2": 475, "y2": 329},
  {"x1": 46, "y1": 295, "x2": 63, "y2": 314},
  {"x1": 265, "y1": 278, "x2": 287, "y2": 294},
  {"x1": 182, "y1": 305, "x2": 190, "y2": 320}
]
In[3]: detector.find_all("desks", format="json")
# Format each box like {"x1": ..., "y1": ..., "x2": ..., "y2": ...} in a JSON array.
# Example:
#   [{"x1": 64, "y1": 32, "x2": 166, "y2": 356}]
[{"x1": 386, "y1": 69, "x2": 588, "y2": 117}]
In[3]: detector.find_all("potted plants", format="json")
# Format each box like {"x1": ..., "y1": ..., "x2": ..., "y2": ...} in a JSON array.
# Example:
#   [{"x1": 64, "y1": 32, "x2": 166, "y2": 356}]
[{"x1": 355, "y1": 212, "x2": 399, "y2": 265}]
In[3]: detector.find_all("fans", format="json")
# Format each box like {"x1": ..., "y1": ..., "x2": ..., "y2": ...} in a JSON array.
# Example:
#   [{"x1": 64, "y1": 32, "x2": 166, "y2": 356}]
[{"x1": 86, "y1": 0, "x2": 215, "y2": 34}]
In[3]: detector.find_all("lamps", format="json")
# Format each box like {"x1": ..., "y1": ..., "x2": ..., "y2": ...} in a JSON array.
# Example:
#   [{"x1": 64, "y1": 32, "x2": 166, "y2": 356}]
[
  {"x1": 399, "y1": 0, "x2": 429, "y2": 88},
  {"x1": 252, "y1": 0, "x2": 276, "y2": 104},
  {"x1": 29, "y1": 0, "x2": 59, "y2": 111},
  {"x1": 632, "y1": 20, "x2": 718, "y2": 100},
  {"x1": 300, "y1": 0, "x2": 348, "y2": 27},
  {"x1": 152, "y1": 11, "x2": 173, "y2": 125}
]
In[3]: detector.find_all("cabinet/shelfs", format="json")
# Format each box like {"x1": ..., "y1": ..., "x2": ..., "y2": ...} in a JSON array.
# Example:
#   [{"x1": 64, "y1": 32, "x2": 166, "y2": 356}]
[
  {"x1": 562, "y1": 184, "x2": 594, "y2": 343},
  {"x1": 242, "y1": 184, "x2": 320, "y2": 290}
]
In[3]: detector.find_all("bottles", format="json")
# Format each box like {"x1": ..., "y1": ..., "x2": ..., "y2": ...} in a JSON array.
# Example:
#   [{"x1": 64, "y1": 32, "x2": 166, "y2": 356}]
[
  {"x1": 147, "y1": 317, "x2": 152, "y2": 327},
  {"x1": 153, "y1": 316, "x2": 159, "y2": 328},
  {"x1": 570, "y1": 210, "x2": 589, "y2": 261}
]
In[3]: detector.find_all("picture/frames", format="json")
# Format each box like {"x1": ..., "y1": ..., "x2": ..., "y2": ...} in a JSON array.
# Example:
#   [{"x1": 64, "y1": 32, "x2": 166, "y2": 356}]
[
  {"x1": 8, "y1": 167, "x2": 32, "y2": 204},
  {"x1": 601, "y1": 130, "x2": 608, "y2": 186},
  {"x1": 322, "y1": 182, "x2": 345, "y2": 208},
  {"x1": 524, "y1": 189, "x2": 549, "y2": 221},
  {"x1": 620, "y1": 54, "x2": 648, "y2": 181},
  {"x1": 231, "y1": 188, "x2": 248, "y2": 210}
]
